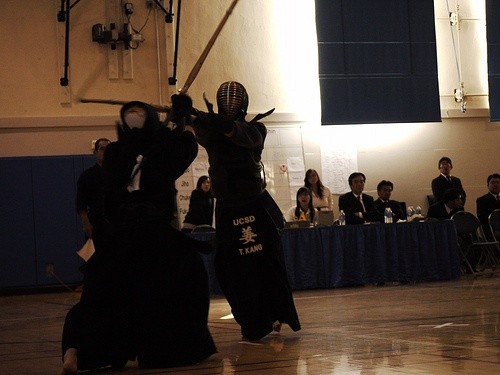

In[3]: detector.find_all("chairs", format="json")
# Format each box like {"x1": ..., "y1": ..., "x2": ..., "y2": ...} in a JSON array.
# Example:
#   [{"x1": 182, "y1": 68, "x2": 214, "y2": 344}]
[{"x1": 450, "y1": 208, "x2": 500, "y2": 280}]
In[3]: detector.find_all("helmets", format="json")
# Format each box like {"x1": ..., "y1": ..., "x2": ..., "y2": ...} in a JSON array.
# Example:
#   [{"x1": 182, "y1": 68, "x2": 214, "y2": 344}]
[{"x1": 217, "y1": 82, "x2": 248, "y2": 121}]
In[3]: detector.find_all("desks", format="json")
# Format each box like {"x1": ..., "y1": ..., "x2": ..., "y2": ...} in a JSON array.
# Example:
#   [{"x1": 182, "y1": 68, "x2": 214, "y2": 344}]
[{"x1": 185, "y1": 219, "x2": 475, "y2": 292}]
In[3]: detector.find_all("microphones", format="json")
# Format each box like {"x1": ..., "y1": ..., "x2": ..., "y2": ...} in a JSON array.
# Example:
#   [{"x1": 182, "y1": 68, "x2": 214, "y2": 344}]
[{"x1": 317, "y1": 207, "x2": 320, "y2": 212}]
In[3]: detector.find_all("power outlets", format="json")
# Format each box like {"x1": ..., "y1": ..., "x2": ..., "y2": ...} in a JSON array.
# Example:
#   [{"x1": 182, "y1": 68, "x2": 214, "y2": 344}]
[{"x1": 91, "y1": 139, "x2": 97, "y2": 149}]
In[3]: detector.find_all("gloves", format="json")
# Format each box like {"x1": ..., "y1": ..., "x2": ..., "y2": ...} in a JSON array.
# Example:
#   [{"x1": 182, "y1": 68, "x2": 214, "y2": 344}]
[{"x1": 169, "y1": 93, "x2": 192, "y2": 125}]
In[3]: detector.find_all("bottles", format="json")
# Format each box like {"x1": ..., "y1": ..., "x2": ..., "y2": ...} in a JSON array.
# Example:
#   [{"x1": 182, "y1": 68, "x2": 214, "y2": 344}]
[
  {"x1": 415, "y1": 204, "x2": 422, "y2": 213},
  {"x1": 339, "y1": 209, "x2": 346, "y2": 226},
  {"x1": 407, "y1": 205, "x2": 415, "y2": 219},
  {"x1": 385, "y1": 207, "x2": 392, "y2": 224}
]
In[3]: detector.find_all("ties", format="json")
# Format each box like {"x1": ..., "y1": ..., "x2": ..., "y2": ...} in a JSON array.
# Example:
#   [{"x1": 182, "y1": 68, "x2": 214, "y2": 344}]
[
  {"x1": 357, "y1": 195, "x2": 361, "y2": 203},
  {"x1": 446, "y1": 176, "x2": 451, "y2": 183},
  {"x1": 496, "y1": 195, "x2": 499, "y2": 200}
]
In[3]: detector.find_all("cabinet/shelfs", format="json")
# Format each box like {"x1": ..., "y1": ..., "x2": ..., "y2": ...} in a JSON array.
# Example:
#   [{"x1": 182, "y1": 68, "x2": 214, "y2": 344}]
[{"x1": 0, "y1": 154, "x2": 97, "y2": 294}]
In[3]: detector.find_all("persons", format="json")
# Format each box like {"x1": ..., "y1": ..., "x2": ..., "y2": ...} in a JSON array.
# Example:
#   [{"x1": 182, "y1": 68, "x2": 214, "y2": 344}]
[
  {"x1": 374, "y1": 180, "x2": 407, "y2": 224},
  {"x1": 96, "y1": 93, "x2": 198, "y2": 227},
  {"x1": 476, "y1": 174, "x2": 500, "y2": 241},
  {"x1": 283, "y1": 187, "x2": 316, "y2": 224},
  {"x1": 431, "y1": 156, "x2": 466, "y2": 206},
  {"x1": 185, "y1": 175, "x2": 214, "y2": 227},
  {"x1": 170, "y1": 82, "x2": 302, "y2": 340},
  {"x1": 62, "y1": 165, "x2": 217, "y2": 375},
  {"x1": 304, "y1": 168, "x2": 333, "y2": 212},
  {"x1": 338, "y1": 172, "x2": 380, "y2": 224},
  {"x1": 427, "y1": 190, "x2": 462, "y2": 219},
  {"x1": 78, "y1": 138, "x2": 112, "y2": 298}
]
